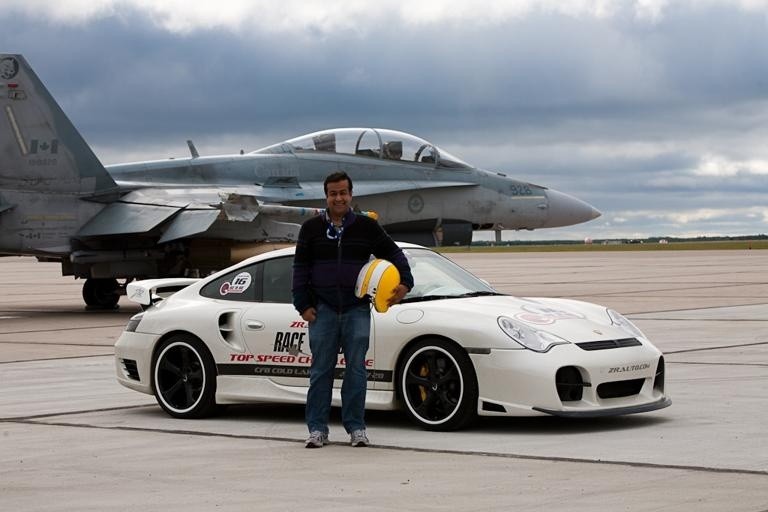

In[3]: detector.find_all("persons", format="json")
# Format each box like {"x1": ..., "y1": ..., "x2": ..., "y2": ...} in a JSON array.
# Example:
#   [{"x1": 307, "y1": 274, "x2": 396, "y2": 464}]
[{"x1": 288, "y1": 170, "x2": 416, "y2": 448}]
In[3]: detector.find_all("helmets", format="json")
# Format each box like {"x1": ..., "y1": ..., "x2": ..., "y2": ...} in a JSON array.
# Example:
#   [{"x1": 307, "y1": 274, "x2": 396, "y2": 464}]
[{"x1": 354, "y1": 258, "x2": 400, "y2": 313}]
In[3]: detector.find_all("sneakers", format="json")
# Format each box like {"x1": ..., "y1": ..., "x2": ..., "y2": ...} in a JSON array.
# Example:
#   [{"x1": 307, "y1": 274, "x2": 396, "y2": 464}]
[
  {"x1": 304, "y1": 431, "x2": 329, "y2": 448},
  {"x1": 350, "y1": 429, "x2": 369, "y2": 447}
]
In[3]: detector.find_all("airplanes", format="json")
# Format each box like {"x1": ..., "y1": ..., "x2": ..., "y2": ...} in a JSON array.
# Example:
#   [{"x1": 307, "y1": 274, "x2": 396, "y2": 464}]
[{"x1": 0, "y1": 47, "x2": 602, "y2": 311}]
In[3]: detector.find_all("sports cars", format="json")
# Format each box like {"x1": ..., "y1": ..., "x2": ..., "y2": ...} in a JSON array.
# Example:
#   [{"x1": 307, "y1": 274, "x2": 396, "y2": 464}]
[{"x1": 111, "y1": 241, "x2": 677, "y2": 429}]
